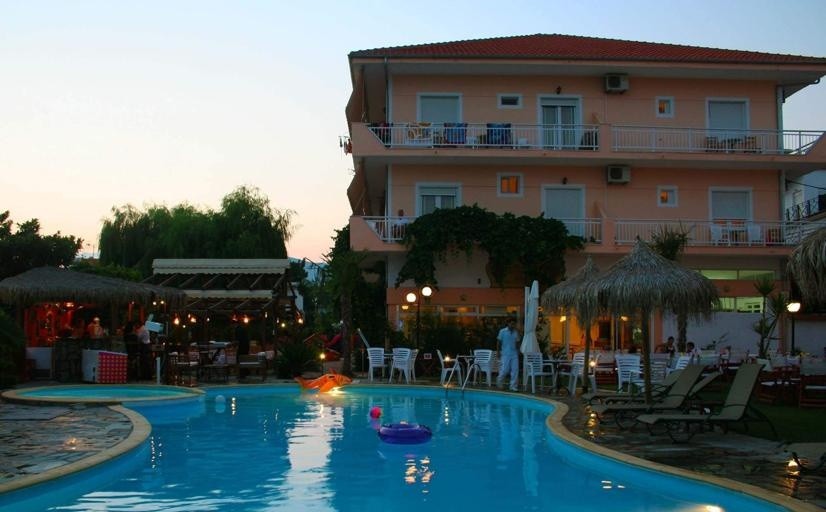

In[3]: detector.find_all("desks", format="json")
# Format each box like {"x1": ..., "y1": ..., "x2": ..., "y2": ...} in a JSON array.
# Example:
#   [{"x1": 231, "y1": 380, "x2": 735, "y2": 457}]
[{"x1": 722, "y1": 138, "x2": 743, "y2": 152}]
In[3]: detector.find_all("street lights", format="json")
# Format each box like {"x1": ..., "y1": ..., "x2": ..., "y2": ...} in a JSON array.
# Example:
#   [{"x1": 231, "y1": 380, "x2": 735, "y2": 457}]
[
  {"x1": 786, "y1": 275, "x2": 804, "y2": 358},
  {"x1": 405, "y1": 265, "x2": 433, "y2": 376}
]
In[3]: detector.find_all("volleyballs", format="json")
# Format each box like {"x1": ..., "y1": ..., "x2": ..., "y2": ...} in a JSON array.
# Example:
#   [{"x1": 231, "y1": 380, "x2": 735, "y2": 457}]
[{"x1": 370, "y1": 407, "x2": 380, "y2": 419}]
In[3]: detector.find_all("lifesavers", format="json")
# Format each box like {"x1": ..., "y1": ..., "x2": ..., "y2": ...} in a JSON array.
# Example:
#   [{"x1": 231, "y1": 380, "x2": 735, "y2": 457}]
[{"x1": 379, "y1": 424, "x2": 431, "y2": 444}]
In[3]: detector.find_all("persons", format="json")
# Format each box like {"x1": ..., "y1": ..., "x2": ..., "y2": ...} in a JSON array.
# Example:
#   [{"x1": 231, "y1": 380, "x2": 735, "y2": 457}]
[
  {"x1": 655, "y1": 336, "x2": 676, "y2": 357},
  {"x1": 213, "y1": 340, "x2": 243, "y2": 361},
  {"x1": 133, "y1": 320, "x2": 152, "y2": 380},
  {"x1": 496, "y1": 318, "x2": 521, "y2": 392},
  {"x1": 686, "y1": 342, "x2": 694, "y2": 352},
  {"x1": 123, "y1": 321, "x2": 149, "y2": 382}
]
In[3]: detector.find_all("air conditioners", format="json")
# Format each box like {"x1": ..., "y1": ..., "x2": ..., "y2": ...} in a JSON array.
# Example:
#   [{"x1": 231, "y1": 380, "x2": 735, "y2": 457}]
[
  {"x1": 607, "y1": 166, "x2": 632, "y2": 184},
  {"x1": 604, "y1": 73, "x2": 630, "y2": 93}
]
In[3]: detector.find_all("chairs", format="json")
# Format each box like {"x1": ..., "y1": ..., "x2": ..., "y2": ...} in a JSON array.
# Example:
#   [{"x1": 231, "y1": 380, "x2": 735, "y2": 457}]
[
  {"x1": 436, "y1": 348, "x2": 603, "y2": 398},
  {"x1": 705, "y1": 136, "x2": 720, "y2": 152},
  {"x1": 615, "y1": 348, "x2": 826, "y2": 409},
  {"x1": 365, "y1": 346, "x2": 419, "y2": 385},
  {"x1": 690, "y1": 216, "x2": 767, "y2": 247},
  {"x1": 583, "y1": 364, "x2": 784, "y2": 447},
  {"x1": 157, "y1": 339, "x2": 270, "y2": 389},
  {"x1": 744, "y1": 135, "x2": 762, "y2": 153}
]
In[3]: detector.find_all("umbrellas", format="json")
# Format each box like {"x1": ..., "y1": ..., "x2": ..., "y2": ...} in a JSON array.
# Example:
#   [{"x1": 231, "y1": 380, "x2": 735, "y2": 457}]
[{"x1": 519, "y1": 280, "x2": 542, "y2": 354}]
